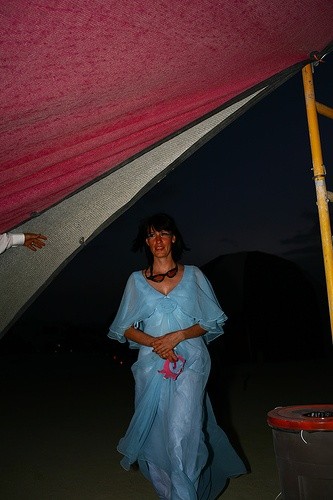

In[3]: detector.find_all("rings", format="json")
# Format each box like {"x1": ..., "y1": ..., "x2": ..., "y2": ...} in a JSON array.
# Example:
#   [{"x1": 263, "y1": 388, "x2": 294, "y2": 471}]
[{"x1": 32, "y1": 242, "x2": 34, "y2": 245}]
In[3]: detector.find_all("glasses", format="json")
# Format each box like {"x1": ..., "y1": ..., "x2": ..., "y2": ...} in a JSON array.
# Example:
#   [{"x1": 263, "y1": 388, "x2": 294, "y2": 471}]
[{"x1": 145, "y1": 261, "x2": 178, "y2": 282}]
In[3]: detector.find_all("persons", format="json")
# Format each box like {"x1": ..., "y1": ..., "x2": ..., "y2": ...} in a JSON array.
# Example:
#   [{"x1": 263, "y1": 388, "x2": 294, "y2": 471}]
[
  {"x1": 108, "y1": 214, "x2": 247, "y2": 500},
  {"x1": 0, "y1": 232, "x2": 47, "y2": 254}
]
